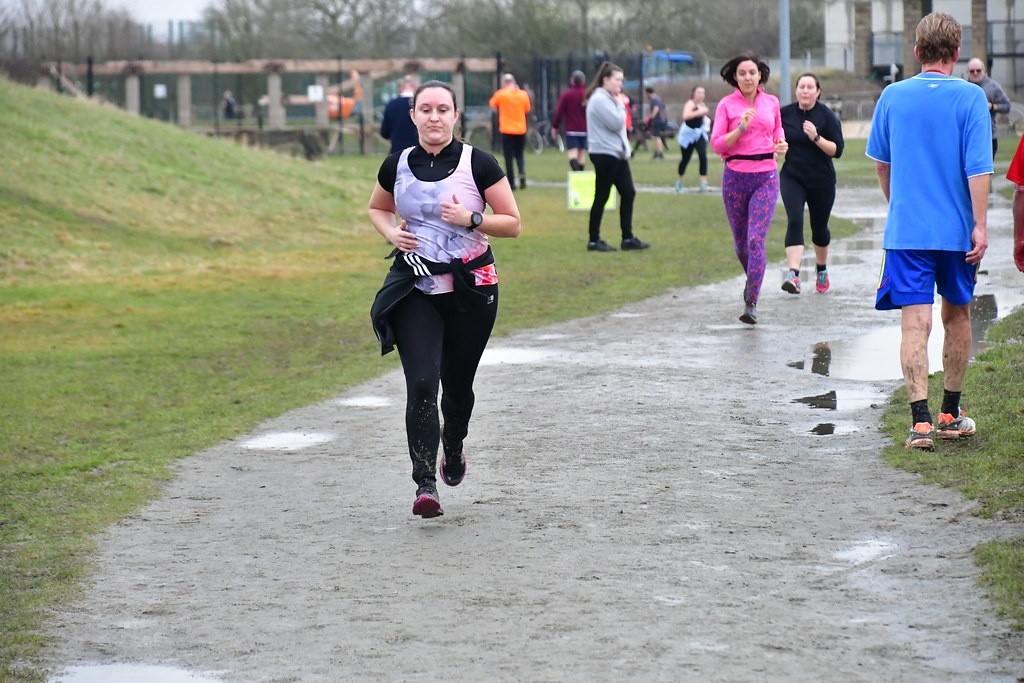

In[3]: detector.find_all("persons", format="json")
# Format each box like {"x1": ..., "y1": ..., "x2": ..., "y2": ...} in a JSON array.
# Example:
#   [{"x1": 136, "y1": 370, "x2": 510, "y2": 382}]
[
  {"x1": 223, "y1": 90, "x2": 239, "y2": 118},
  {"x1": 1005, "y1": 133, "x2": 1024, "y2": 273},
  {"x1": 379, "y1": 74, "x2": 419, "y2": 154},
  {"x1": 675, "y1": 84, "x2": 709, "y2": 193},
  {"x1": 643, "y1": 86, "x2": 670, "y2": 161},
  {"x1": 966, "y1": 57, "x2": 1012, "y2": 193},
  {"x1": 489, "y1": 73, "x2": 530, "y2": 189},
  {"x1": 710, "y1": 54, "x2": 788, "y2": 325},
  {"x1": 327, "y1": 69, "x2": 365, "y2": 120},
  {"x1": 581, "y1": 61, "x2": 652, "y2": 252},
  {"x1": 864, "y1": 11, "x2": 996, "y2": 448},
  {"x1": 551, "y1": 70, "x2": 589, "y2": 170},
  {"x1": 368, "y1": 79, "x2": 523, "y2": 519},
  {"x1": 778, "y1": 73, "x2": 843, "y2": 295}
]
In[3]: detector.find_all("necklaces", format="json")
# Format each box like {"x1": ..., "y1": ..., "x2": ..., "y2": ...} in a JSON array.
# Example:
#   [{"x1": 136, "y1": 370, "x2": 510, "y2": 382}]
[{"x1": 925, "y1": 70, "x2": 946, "y2": 75}]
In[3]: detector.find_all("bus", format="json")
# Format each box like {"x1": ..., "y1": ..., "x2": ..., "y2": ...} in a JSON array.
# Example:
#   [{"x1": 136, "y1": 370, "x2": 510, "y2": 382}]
[{"x1": 537, "y1": 46, "x2": 696, "y2": 138}]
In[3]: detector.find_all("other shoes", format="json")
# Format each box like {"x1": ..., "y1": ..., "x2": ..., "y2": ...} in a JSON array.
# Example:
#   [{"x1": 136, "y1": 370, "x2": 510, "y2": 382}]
[
  {"x1": 676, "y1": 182, "x2": 681, "y2": 191},
  {"x1": 700, "y1": 184, "x2": 711, "y2": 192}
]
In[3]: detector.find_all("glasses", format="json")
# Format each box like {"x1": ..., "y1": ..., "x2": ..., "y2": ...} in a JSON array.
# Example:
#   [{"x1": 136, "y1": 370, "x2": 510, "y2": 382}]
[{"x1": 969, "y1": 69, "x2": 982, "y2": 73}]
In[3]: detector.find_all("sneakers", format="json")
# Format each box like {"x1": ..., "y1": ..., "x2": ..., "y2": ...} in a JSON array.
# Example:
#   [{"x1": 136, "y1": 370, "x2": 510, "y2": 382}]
[
  {"x1": 412, "y1": 489, "x2": 443, "y2": 519},
  {"x1": 740, "y1": 302, "x2": 756, "y2": 324},
  {"x1": 439, "y1": 424, "x2": 467, "y2": 486},
  {"x1": 936, "y1": 407, "x2": 976, "y2": 438},
  {"x1": 815, "y1": 263, "x2": 829, "y2": 293},
  {"x1": 781, "y1": 270, "x2": 801, "y2": 294},
  {"x1": 587, "y1": 240, "x2": 616, "y2": 252},
  {"x1": 904, "y1": 422, "x2": 934, "y2": 451},
  {"x1": 621, "y1": 238, "x2": 649, "y2": 250}
]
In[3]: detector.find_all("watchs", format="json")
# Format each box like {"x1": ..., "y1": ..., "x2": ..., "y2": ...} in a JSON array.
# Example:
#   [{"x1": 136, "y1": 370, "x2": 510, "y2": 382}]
[{"x1": 466, "y1": 211, "x2": 483, "y2": 233}]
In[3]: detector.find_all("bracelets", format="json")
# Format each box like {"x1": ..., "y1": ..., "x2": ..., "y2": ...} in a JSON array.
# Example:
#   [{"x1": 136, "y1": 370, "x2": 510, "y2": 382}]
[
  {"x1": 991, "y1": 103, "x2": 994, "y2": 110},
  {"x1": 738, "y1": 124, "x2": 745, "y2": 133},
  {"x1": 813, "y1": 134, "x2": 820, "y2": 143}
]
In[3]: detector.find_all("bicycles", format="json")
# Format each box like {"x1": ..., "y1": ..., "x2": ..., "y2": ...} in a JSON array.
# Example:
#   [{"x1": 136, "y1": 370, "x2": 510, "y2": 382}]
[{"x1": 527, "y1": 116, "x2": 563, "y2": 155}]
